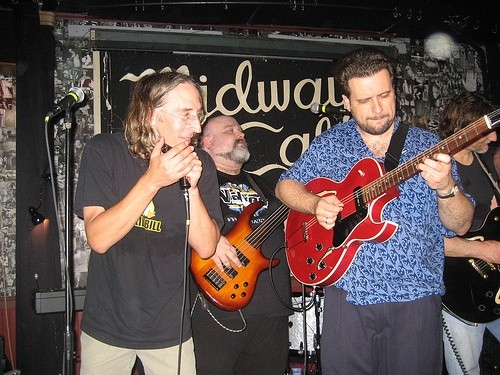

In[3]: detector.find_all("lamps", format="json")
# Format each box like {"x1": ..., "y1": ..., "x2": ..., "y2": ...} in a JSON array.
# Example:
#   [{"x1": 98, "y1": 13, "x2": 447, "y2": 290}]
[{"x1": 28, "y1": 172, "x2": 54, "y2": 226}]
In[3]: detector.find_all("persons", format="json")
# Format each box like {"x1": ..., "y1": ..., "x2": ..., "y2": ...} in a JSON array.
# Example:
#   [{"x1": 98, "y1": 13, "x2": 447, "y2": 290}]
[
  {"x1": 274, "y1": 52, "x2": 474, "y2": 375},
  {"x1": 188, "y1": 115, "x2": 295, "y2": 375},
  {"x1": 74, "y1": 73, "x2": 225, "y2": 375},
  {"x1": 442, "y1": 92, "x2": 500, "y2": 375}
]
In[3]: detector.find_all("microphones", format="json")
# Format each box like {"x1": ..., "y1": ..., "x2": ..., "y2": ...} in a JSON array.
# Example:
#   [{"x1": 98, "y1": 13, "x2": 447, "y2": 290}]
[
  {"x1": 45, "y1": 86, "x2": 85, "y2": 120},
  {"x1": 179, "y1": 177, "x2": 192, "y2": 204}
]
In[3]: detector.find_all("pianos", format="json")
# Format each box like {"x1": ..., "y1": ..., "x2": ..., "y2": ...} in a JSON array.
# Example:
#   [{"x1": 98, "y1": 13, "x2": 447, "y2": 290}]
[{"x1": 33, "y1": 287, "x2": 87, "y2": 316}]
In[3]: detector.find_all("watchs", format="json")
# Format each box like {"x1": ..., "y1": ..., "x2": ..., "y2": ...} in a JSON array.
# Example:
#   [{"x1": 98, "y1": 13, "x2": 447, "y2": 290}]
[{"x1": 436, "y1": 184, "x2": 459, "y2": 199}]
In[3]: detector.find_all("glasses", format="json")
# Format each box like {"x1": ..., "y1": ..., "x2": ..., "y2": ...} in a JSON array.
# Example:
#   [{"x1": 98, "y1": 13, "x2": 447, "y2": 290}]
[{"x1": 155, "y1": 108, "x2": 204, "y2": 125}]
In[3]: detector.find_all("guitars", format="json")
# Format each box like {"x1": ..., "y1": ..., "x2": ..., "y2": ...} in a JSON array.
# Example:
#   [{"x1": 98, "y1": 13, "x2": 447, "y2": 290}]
[
  {"x1": 190, "y1": 200, "x2": 289, "y2": 312},
  {"x1": 440, "y1": 206, "x2": 500, "y2": 325},
  {"x1": 284, "y1": 106, "x2": 500, "y2": 289}
]
